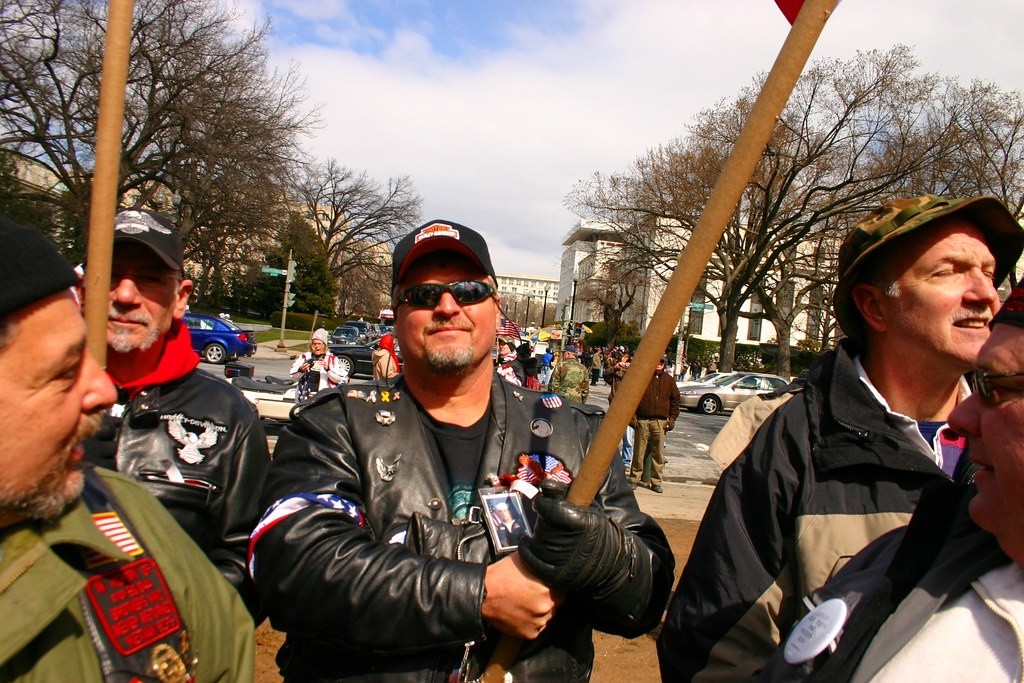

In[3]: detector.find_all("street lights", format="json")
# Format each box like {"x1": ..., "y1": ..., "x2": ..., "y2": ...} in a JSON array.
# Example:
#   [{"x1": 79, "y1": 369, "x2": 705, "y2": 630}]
[
  {"x1": 540, "y1": 284, "x2": 552, "y2": 328},
  {"x1": 513, "y1": 299, "x2": 519, "y2": 324},
  {"x1": 504, "y1": 300, "x2": 509, "y2": 316},
  {"x1": 523, "y1": 291, "x2": 532, "y2": 336},
  {"x1": 568, "y1": 274, "x2": 579, "y2": 325}
]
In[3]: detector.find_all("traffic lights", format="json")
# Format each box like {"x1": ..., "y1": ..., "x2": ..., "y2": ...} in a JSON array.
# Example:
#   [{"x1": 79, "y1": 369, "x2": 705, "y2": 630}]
[
  {"x1": 286, "y1": 292, "x2": 297, "y2": 309},
  {"x1": 573, "y1": 323, "x2": 582, "y2": 338},
  {"x1": 566, "y1": 322, "x2": 574, "y2": 338}
]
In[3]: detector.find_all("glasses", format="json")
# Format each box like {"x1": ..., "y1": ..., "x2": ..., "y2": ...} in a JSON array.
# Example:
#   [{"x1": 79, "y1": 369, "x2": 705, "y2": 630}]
[
  {"x1": 971, "y1": 367, "x2": 1024, "y2": 405},
  {"x1": 397, "y1": 281, "x2": 498, "y2": 309}
]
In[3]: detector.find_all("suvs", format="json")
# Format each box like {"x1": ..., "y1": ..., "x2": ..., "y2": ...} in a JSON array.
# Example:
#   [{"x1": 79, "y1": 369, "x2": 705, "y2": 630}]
[{"x1": 345, "y1": 320, "x2": 373, "y2": 344}]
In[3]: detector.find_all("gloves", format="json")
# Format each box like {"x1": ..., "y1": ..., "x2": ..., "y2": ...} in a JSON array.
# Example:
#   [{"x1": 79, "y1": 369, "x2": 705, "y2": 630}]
[
  {"x1": 665, "y1": 416, "x2": 674, "y2": 431},
  {"x1": 628, "y1": 413, "x2": 637, "y2": 429},
  {"x1": 519, "y1": 481, "x2": 640, "y2": 599}
]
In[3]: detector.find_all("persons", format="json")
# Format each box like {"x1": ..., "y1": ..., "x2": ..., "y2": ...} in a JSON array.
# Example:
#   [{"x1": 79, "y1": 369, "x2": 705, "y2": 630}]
[
  {"x1": 656, "y1": 192, "x2": 1023, "y2": 683},
  {"x1": 494, "y1": 336, "x2": 590, "y2": 405},
  {"x1": 582, "y1": 343, "x2": 634, "y2": 407},
  {"x1": 83, "y1": 208, "x2": 270, "y2": 594},
  {"x1": 0, "y1": 213, "x2": 257, "y2": 683},
  {"x1": 371, "y1": 334, "x2": 401, "y2": 380},
  {"x1": 247, "y1": 218, "x2": 677, "y2": 682},
  {"x1": 494, "y1": 502, "x2": 530, "y2": 547},
  {"x1": 621, "y1": 425, "x2": 634, "y2": 472},
  {"x1": 628, "y1": 355, "x2": 681, "y2": 493},
  {"x1": 663, "y1": 354, "x2": 717, "y2": 382},
  {"x1": 287, "y1": 327, "x2": 345, "y2": 404}
]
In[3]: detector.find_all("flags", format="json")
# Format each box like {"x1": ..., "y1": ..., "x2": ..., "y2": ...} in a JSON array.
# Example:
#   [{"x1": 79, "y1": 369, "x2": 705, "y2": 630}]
[
  {"x1": 487, "y1": 498, "x2": 502, "y2": 532},
  {"x1": 498, "y1": 319, "x2": 522, "y2": 341}
]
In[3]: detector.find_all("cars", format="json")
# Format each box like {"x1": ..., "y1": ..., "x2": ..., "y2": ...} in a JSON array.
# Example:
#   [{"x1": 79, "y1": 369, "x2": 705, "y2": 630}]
[
  {"x1": 182, "y1": 311, "x2": 257, "y2": 365},
  {"x1": 385, "y1": 326, "x2": 395, "y2": 334},
  {"x1": 377, "y1": 323, "x2": 387, "y2": 335},
  {"x1": 333, "y1": 326, "x2": 361, "y2": 349},
  {"x1": 328, "y1": 338, "x2": 405, "y2": 379},
  {"x1": 370, "y1": 324, "x2": 382, "y2": 340},
  {"x1": 674, "y1": 373, "x2": 776, "y2": 391},
  {"x1": 675, "y1": 372, "x2": 792, "y2": 414}
]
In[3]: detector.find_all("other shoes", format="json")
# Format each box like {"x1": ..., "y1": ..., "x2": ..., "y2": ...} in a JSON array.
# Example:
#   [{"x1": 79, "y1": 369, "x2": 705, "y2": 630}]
[
  {"x1": 651, "y1": 484, "x2": 662, "y2": 492},
  {"x1": 627, "y1": 480, "x2": 636, "y2": 490}
]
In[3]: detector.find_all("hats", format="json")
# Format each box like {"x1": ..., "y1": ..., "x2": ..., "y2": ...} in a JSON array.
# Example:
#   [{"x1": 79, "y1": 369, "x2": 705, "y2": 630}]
[
  {"x1": 392, "y1": 220, "x2": 498, "y2": 295},
  {"x1": 312, "y1": 328, "x2": 328, "y2": 346},
  {"x1": 833, "y1": 195, "x2": 1024, "y2": 338},
  {"x1": 559, "y1": 346, "x2": 578, "y2": 354},
  {"x1": 495, "y1": 335, "x2": 515, "y2": 348},
  {"x1": 112, "y1": 207, "x2": 186, "y2": 282},
  {"x1": 0, "y1": 217, "x2": 79, "y2": 318},
  {"x1": 661, "y1": 355, "x2": 667, "y2": 362},
  {"x1": 987, "y1": 277, "x2": 1024, "y2": 328}
]
